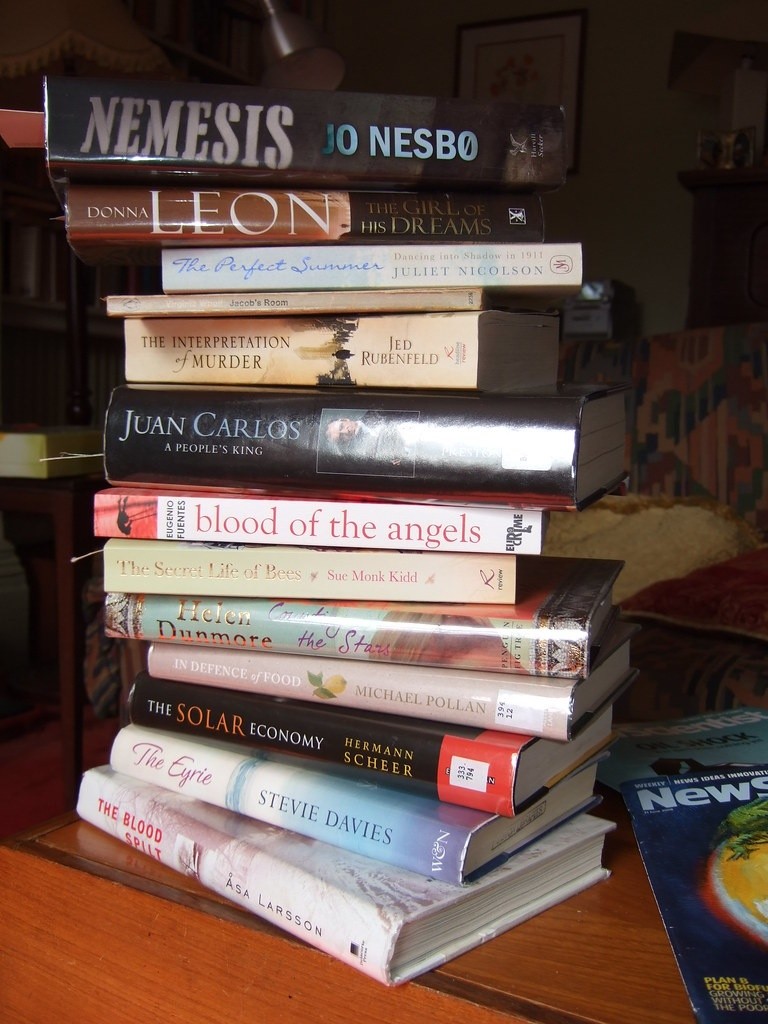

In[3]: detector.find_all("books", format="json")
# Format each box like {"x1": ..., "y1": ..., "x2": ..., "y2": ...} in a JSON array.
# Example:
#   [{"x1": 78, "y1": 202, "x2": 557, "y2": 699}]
[
  {"x1": 45, "y1": 74, "x2": 644, "y2": 988},
  {"x1": 594, "y1": 704, "x2": 768, "y2": 1024},
  {"x1": 0, "y1": 221, "x2": 161, "y2": 312},
  {"x1": 122, "y1": 0, "x2": 263, "y2": 80}
]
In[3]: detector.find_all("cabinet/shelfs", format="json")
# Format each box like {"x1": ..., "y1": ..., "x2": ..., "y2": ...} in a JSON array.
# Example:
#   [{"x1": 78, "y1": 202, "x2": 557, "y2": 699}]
[
  {"x1": 676, "y1": 166, "x2": 768, "y2": 329},
  {"x1": 0, "y1": 0, "x2": 314, "y2": 839}
]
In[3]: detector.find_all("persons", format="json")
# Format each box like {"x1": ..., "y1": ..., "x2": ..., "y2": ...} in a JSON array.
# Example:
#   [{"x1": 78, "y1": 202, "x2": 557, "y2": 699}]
[{"x1": 323, "y1": 411, "x2": 415, "y2": 466}]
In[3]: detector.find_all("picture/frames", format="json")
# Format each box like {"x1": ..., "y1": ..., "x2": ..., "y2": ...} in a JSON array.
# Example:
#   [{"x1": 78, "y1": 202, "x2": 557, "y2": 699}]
[{"x1": 451, "y1": 7, "x2": 591, "y2": 175}]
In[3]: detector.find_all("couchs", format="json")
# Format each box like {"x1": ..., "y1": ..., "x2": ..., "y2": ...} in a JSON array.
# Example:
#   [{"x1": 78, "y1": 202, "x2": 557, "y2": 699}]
[{"x1": 564, "y1": 322, "x2": 768, "y2": 544}]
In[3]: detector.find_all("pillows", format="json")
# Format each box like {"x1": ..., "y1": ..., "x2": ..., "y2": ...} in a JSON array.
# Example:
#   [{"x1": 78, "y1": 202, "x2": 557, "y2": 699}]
[{"x1": 617, "y1": 545, "x2": 768, "y2": 643}]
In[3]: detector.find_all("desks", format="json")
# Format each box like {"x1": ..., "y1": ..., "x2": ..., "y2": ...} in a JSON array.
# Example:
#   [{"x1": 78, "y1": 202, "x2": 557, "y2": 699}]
[
  {"x1": 0, "y1": 784, "x2": 704, "y2": 1024},
  {"x1": 0, "y1": 476, "x2": 111, "y2": 813}
]
